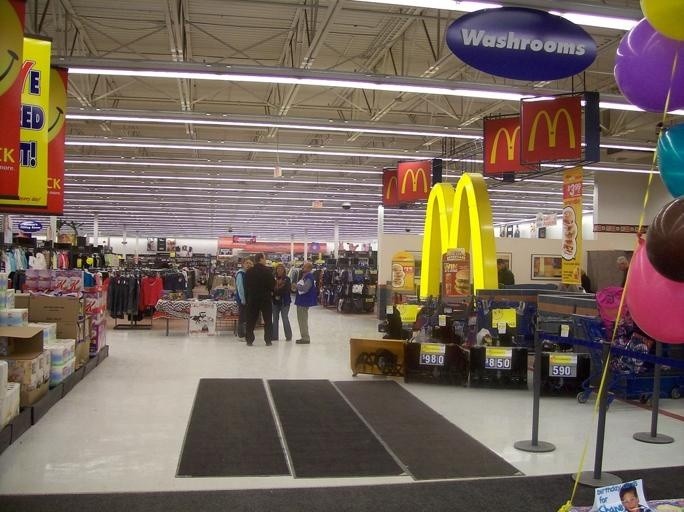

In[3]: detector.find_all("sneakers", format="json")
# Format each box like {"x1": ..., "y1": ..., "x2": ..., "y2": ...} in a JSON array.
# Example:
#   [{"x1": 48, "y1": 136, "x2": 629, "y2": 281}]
[
  {"x1": 296, "y1": 340, "x2": 309, "y2": 344},
  {"x1": 238, "y1": 337, "x2": 246, "y2": 342}
]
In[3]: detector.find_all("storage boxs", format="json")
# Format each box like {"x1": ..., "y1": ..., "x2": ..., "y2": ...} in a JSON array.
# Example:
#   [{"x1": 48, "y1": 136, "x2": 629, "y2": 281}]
[{"x1": 0, "y1": 273, "x2": 89, "y2": 431}]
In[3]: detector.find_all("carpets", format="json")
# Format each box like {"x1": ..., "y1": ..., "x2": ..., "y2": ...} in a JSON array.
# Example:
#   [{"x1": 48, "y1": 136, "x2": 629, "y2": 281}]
[
  {"x1": 332, "y1": 379, "x2": 526, "y2": 480},
  {"x1": 175, "y1": 377, "x2": 291, "y2": 478},
  {"x1": 267, "y1": 379, "x2": 407, "y2": 479}
]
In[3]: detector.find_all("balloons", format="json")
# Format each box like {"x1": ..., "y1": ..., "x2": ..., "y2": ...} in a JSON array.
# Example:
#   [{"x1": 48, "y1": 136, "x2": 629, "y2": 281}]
[
  {"x1": 637, "y1": 0, "x2": 684, "y2": 41},
  {"x1": 613, "y1": 17, "x2": 684, "y2": 113},
  {"x1": 656, "y1": 123, "x2": 684, "y2": 198},
  {"x1": 627, "y1": 245, "x2": 684, "y2": 344},
  {"x1": 646, "y1": 194, "x2": 684, "y2": 283}
]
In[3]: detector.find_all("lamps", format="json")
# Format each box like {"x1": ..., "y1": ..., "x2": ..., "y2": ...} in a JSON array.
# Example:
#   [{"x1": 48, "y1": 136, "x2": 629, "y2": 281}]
[
  {"x1": 64, "y1": 153, "x2": 594, "y2": 186},
  {"x1": 65, "y1": 108, "x2": 658, "y2": 153},
  {"x1": 50, "y1": 54, "x2": 684, "y2": 116},
  {"x1": 65, "y1": 133, "x2": 660, "y2": 173},
  {"x1": 407, "y1": 2, "x2": 642, "y2": 31},
  {"x1": 312, "y1": 152, "x2": 323, "y2": 209},
  {"x1": 273, "y1": 127, "x2": 282, "y2": 177}
]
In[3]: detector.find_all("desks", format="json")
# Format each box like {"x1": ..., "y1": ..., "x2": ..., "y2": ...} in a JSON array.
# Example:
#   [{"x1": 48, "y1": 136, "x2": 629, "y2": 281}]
[{"x1": 155, "y1": 299, "x2": 239, "y2": 334}]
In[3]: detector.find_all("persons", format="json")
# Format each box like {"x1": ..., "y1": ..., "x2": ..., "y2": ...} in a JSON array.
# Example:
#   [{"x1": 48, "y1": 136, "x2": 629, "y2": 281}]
[
  {"x1": 272, "y1": 264, "x2": 293, "y2": 341},
  {"x1": 497, "y1": 258, "x2": 515, "y2": 288},
  {"x1": 581, "y1": 269, "x2": 592, "y2": 293},
  {"x1": 617, "y1": 256, "x2": 630, "y2": 288},
  {"x1": 294, "y1": 262, "x2": 318, "y2": 345},
  {"x1": 244, "y1": 254, "x2": 273, "y2": 346},
  {"x1": 234, "y1": 258, "x2": 252, "y2": 338},
  {"x1": 619, "y1": 482, "x2": 652, "y2": 512}
]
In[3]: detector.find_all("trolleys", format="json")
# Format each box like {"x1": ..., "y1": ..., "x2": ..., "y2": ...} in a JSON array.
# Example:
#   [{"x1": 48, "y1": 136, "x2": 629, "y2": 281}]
[{"x1": 576, "y1": 318, "x2": 683, "y2": 412}]
